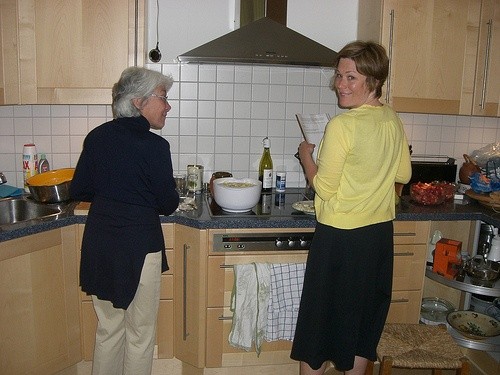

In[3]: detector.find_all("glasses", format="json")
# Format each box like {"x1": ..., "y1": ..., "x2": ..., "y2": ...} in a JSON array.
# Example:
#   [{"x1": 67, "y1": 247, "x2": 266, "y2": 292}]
[{"x1": 148, "y1": 93, "x2": 169, "y2": 102}]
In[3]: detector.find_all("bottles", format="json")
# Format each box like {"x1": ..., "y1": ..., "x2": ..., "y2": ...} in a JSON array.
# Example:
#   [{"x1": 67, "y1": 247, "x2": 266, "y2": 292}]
[
  {"x1": 257, "y1": 192, "x2": 272, "y2": 219},
  {"x1": 275, "y1": 171, "x2": 286, "y2": 192},
  {"x1": 259, "y1": 138, "x2": 273, "y2": 192},
  {"x1": 174, "y1": 164, "x2": 204, "y2": 198},
  {"x1": 275, "y1": 193, "x2": 285, "y2": 207},
  {"x1": 23, "y1": 143, "x2": 49, "y2": 192}
]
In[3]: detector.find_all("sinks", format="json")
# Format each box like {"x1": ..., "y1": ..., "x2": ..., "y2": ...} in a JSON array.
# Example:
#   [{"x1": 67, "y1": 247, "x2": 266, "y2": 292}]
[{"x1": 0, "y1": 200, "x2": 63, "y2": 226}]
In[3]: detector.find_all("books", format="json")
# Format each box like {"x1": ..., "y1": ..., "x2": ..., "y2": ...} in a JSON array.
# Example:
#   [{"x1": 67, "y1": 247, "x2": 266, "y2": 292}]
[{"x1": 295, "y1": 113, "x2": 332, "y2": 164}]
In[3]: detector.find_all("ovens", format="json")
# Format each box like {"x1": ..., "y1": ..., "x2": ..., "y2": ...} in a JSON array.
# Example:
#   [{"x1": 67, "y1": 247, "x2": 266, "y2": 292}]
[{"x1": 208, "y1": 228, "x2": 329, "y2": 372}]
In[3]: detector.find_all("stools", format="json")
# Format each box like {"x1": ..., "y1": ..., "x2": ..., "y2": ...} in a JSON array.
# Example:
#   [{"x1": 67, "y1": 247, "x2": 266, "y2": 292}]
[{"x1": 376, "y1": 323, "x2": 469, "y2": 375}]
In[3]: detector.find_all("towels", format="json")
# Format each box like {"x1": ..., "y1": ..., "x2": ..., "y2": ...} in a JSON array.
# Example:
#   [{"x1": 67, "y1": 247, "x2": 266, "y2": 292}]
[
  {"x1": 227, "y1": 261, "x2": 271, "y2": 358},
  {"x1": 266, "y1": 262, "x2": 306, "y2": 344}
]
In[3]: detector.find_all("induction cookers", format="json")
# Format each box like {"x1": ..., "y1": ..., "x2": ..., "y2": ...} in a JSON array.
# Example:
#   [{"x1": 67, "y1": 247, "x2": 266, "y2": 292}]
[{"x1": 202, "y1": 181, "x2": 313, "y2": 218}]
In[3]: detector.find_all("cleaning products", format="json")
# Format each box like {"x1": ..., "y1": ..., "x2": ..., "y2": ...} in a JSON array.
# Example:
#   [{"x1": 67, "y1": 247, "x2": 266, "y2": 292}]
[
  {"x1": 38, "y1": 154, "x2": 50, "y2": 174},
  {"x1": 22, "y1": 143, "x2": 38, "y2": 193}
]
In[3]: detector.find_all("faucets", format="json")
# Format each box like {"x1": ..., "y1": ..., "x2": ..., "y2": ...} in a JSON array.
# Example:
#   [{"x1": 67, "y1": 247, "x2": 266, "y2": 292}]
[{"x1": 0, "y1": 172, "x2": 7, "y2": 185}]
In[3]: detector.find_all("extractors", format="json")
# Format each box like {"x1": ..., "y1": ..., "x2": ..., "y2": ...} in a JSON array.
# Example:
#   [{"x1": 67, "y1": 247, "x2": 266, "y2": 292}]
[{"x1": 175, "y1": 0, "x2": 345, "y2": 70}]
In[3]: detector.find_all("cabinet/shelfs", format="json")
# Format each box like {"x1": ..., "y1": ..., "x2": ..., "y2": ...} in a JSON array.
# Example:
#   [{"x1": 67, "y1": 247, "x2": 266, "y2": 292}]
[
  {"x1": 357, "y1": 0, "x2": 500, "y2": 118},
  {"x1": 387, "y1": 221, "x2": 472, "y2": 323},
  {"x1": 0, "y1": 223, "x2": 208, "y2": 375},
  {"x1": 0, "y1": 0, "x2": 145, "y2": 106}
]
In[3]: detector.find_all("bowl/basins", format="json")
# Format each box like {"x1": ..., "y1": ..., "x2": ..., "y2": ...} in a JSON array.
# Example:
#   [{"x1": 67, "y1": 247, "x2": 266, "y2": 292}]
[
  {"x1": 419, "y1": 296, "x2": 500, "y2": 351},
  {"x1": 24, "y1": 166, "x2": 78, "y2": 204},
  {"x1": 212, "y1": 177, "x2": 263, "y2": 213},
  {"x1": 463, "y1": 255, "x2": 500, "y2": 283},
  {"x1": 409, "y1": 182, "x2": 459, "y2": 206}
]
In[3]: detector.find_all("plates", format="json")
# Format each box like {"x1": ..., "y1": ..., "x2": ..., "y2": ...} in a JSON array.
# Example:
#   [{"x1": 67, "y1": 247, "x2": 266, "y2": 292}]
[{"x1": 291, "y1": 200, "x2": 316, "y2": 216}]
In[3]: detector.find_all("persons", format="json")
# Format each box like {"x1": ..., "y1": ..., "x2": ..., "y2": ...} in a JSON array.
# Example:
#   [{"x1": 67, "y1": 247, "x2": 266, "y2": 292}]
[
  {"x1": 70, "y1": 67, "x2": 179, "y2": 375},
  {"x1": 291, "y1": 41, "x2": 412, "y2": 375}
]
